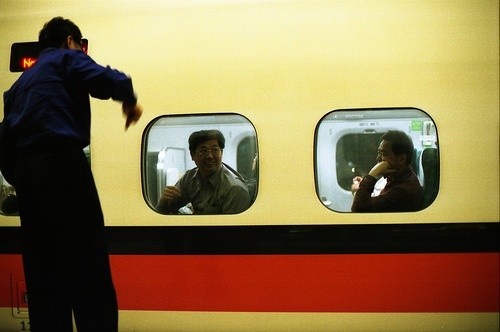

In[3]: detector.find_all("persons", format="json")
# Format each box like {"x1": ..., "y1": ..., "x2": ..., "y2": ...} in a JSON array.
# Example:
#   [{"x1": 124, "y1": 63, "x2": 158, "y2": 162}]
[
  {"x1": 0, "y1": 16, "x2": 144, "y2": 332},
  {"x1": 352, "y1": 176, "x2": 388, "y2": 197},
  {"x1": 155, "y1": 129, "x2": 252, "y2": 215},
  {"x1": 351, "y1": 131, "x2": 424, "y2": 212},
  {"x1": 187, "y1": 153, "x2": 258, "y2": 212}
]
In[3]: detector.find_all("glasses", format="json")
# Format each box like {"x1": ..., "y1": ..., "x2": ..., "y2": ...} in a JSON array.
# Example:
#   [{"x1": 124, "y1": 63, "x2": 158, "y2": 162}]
[{"x1": 195, "y1": 147, "x2": 221, "y2": 155}]
